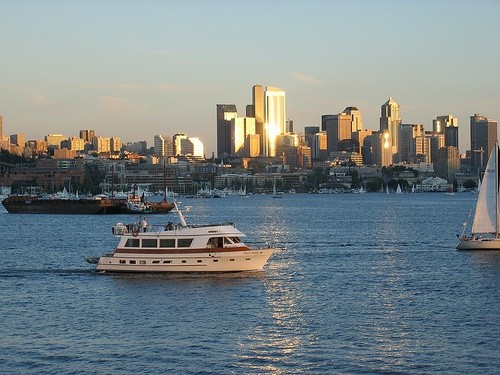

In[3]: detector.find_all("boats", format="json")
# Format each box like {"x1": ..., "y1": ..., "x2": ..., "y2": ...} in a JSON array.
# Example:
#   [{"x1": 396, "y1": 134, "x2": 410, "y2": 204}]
[
  {"x1": 94, "y1": 198, "x2": 276, "y2": 271},
  {"x1": 2, "y1": 158, "x2": 175, "y2": 214}
]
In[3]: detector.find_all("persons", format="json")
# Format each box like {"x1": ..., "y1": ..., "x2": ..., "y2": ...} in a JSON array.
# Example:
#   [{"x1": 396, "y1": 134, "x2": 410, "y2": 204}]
[
  {"x1": 143, "y1": 217, "x2": 148, "y2": 232},
  {"x1": 165, "y1": 221, "x2": 173, "y2": 231}
]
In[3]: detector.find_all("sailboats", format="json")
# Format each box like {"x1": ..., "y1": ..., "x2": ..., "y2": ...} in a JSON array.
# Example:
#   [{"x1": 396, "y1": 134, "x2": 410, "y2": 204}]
[{"x1": 456, "y1": 140, "x2": 500, "y2": 250}]
